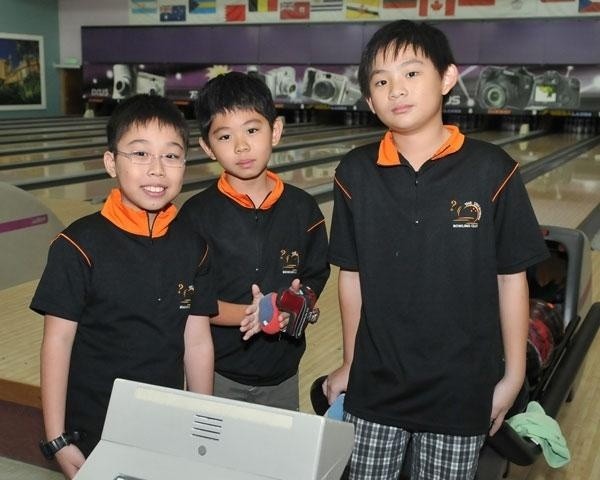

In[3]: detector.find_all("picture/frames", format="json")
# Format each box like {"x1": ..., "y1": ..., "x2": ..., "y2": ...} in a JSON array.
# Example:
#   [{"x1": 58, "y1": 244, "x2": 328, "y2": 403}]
[{"x1": 0, "y1": 32, "x2": 47, "y2": 111}]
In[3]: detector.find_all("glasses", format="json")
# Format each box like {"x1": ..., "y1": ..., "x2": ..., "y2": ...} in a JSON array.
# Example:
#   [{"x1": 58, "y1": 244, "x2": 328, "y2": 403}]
[{"x1": 112, "y1": 150, "x2": 186, "y2": 168}]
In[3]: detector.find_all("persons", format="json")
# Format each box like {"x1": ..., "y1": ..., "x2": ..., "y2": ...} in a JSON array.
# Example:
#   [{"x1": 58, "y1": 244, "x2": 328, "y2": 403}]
[
  {"x1": 173, "y1": 69, "x2": 331, "y2": 415},
  {"x1": 27, "y1": 92, "x2": 221, "y2": 479},
  {"x1": 319, "y1": 14, "x2": 550, "y2": 480}
]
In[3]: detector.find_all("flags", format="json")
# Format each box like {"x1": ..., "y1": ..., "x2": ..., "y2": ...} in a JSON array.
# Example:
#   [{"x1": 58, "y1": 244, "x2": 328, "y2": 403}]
[
  {"x1": 280, "y1": 0, "x2": 310, "y2": 20},
  {"x1": 225, "y1": 4, "x2": 245, "y2": 22},
  {"x1": 578, "y1": 0, "x2": 600, "y2": 14},
  {"x1": 310, "y1": 0, "x2": 343, "y2": 12},
  {"x1": 383, "y1": 0, "x2": 417, "y2": 9},
  {"x1": 458, "y1": 0, "x2": 496, "y2": 6},
  {"x1": 159, "y1": 5, "x2": 186, "y2": 22},
  {"x1": 346, "y1": 0, "x2": 380, "y2": 20},
  {"x1": 419, "y1": 0, "x2": 456, "y2": 17},
  {"x1": 189, "y1": 0, "x2": 216, "y2": 14},
  {"x1": 131, "y1": 0, "x2": 157, "y2": 14},
  {"x1": 248, "y1": 0, "x2": 278, "y2": 13}
]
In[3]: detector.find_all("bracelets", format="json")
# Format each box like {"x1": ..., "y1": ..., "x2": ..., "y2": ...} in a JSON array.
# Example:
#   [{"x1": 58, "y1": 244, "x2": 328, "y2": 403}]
[{"x1": 38, "y1": 430, "x2": 82, "y2": 460}]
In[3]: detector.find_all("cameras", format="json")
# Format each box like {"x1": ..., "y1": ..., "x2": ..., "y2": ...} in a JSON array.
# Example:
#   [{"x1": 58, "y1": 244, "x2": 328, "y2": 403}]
[
  {"x1": 245, "y1": 64, "x2": 300, "y2": 100},
  {"x1": 529, "y1": 66, "x2": 581, "y2": 111},
  {"x1": 300, "y1": 65, "x2": 362, "y2": 106},
  {"x1": 472, "y1": 64, "x2": 534, "y2": 111},
  {"x1": 110, "y1": 64, "x2": 168, "y2": 102}
]
